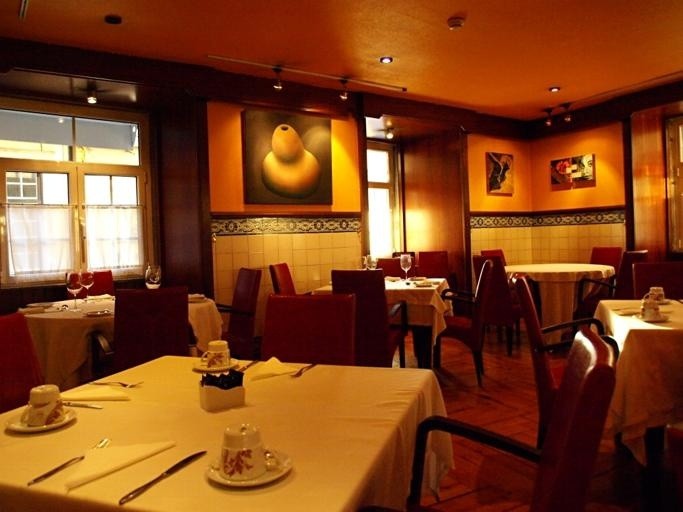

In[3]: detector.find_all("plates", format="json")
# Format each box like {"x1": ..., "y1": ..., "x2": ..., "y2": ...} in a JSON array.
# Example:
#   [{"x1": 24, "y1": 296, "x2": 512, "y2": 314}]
[
  {"x1": 191, "y1": 361, "x2": 238, "y2": 372},
  {"x1": 25, "y1": 302, "x2": 54, "y2": 308},
  {"x1": 84, "y1": 311, "x2": 114, "y2": 317},
  {"x1": 204, "y1": 448, "x2": 293, "y2": 488},
  {"x1": 637, "y1": 316, "x2": 670, "y2": 322},
  {"x1": 3, "y1": 408, "x2": 77, "y2": 435}
]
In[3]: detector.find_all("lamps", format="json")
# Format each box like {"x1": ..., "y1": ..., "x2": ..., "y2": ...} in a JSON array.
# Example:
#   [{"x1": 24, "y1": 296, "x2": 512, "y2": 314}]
[
  {"x1": 272, "y1": 66, "x2": 350, "y2": 101},
  {"x1": 537, "y1": 101, "x2": 576, "y2": 127}
]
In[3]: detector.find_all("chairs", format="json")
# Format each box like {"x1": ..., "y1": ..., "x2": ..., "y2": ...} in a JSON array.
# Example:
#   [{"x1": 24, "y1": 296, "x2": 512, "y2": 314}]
[
  {"x1": 269, "y1": 262, "x2": 296, "y2": 293},
  {"x1": 359, "y1": 329, "x2": 617, "y2": 512},
  {"x1": 588, "y1": 244, "x2": 624, "y2": 294},
  {"x1": 512, "y1": 273, "x2": 627, "y2": 467},
  {"x1": 573, "y1": 249, "x2": 646, "y2": 345},
  {"x1": 375, "y1": 259, "x2": 416, "y2": 276},
  {"x1": 417, "y1": 252, "x2": 458, "y2": 288},
  {"x1": 87, "y1": 285, "x2": 188, "y2": 384},
  {"x1": 261, "y1": 293, "x2": 355, "y2": 366},
  {"x1": 65, "y1": 270, "x2": 114, "y2": 300},
  {"x1": 392, "y1": 252, "x2": 416, "y2": 259},
  {"x1": 631, "y1": 261, "x2": 683, "y2": 301},
  {"x1": 429, "y1": 260, "x2": 492, "y2": 388},
  {"x1": 212, "y1": 266, "x2": 262, "y2": 359},
  {"x1": 0, "y1": 311, "x2": 51, "y2": 405},
  {"x1": 331, "y1": 269, "x2": 412, "y2": 369},
  {"x1": 474, "y1": 255, "x2": 533, "y2": 355},
  {"x1": 482, "y1": 249, "x2": 507, "y2": 265}
]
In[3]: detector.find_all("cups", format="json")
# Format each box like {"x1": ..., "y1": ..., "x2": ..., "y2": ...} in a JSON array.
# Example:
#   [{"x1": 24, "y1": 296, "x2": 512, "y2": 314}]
[
  {"x1": 25, "y1": 384, "x2": 66, "y2": 426},
  {"x1": 143, "y1": 264, "x2": 160, "y2": 289},
  {"x1": 645, "y1": 286, "x2": 666, "y2": 305},
  {"x1": 206, "y1": 339, "x2": 231, "y2": 368},
  {"x1": 357, "y1": 253, "x2": 376, "y2": 270},
  {"x1": 215, "y1": 424, "x2": 264, "y2": 481},
  {"x1": 638, "y1": 298, "x2": 660, "y2": 318}
]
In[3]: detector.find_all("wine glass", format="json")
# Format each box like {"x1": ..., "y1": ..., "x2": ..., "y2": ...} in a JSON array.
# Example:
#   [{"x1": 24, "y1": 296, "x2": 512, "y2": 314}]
[
  {"x1": 400, "y1": 251, "x2": 412, "y2": 284},
  {"x1": 65, "y1": 270, "x2": 83, "y2": 312},
  {"x1": 77, "y1": 267, "x2": 95, "y2": 308}
]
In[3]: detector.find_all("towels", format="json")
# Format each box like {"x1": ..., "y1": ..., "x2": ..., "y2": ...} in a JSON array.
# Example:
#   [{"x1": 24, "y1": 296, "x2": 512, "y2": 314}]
[{"x1": 63, "y1": 439, "x2": 172, "y2": 490}]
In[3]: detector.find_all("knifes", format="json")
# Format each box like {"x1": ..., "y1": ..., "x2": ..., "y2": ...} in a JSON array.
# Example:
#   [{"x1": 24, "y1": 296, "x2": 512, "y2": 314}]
[
  {"x1": 238, "y1": 359, "x2": 258, "y2": 373},
  {"x1": 117, "y1": 448, "x2": 207, "y2": 507},
  {"x1": 60, "y1": 399, "x2": 102, "y2": 412}
]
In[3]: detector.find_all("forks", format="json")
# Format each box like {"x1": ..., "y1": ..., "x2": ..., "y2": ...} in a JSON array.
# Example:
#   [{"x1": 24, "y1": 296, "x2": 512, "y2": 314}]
[
  {"x1": 87, "y1": 380, "x2": 142, "y2": 389},
  {"x1": 290, "y1": 361, "x2": 318, "y2": 378},
  {"x1": 26, "y1": 439, "x2": 109, "y2": 486}
]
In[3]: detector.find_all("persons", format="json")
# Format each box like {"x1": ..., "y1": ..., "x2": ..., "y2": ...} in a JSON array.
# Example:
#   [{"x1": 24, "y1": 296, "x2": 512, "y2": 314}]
[{"x1": 575, "y1": 154, "x2": 594, "y2": 182}]
